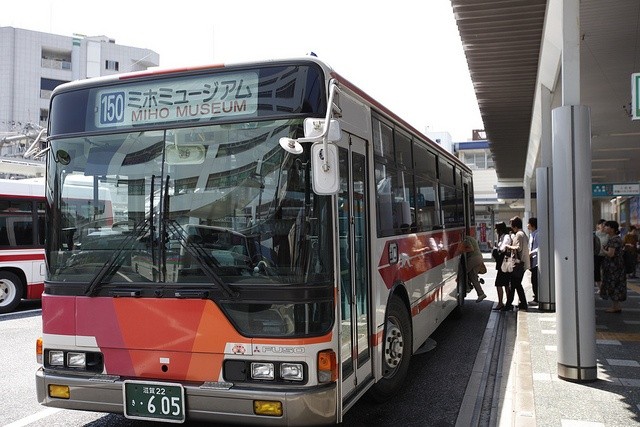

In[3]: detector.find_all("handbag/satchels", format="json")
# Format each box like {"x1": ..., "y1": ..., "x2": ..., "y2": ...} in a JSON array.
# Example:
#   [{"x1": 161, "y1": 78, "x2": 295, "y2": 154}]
[{"x1": 500, "y1": 247, "x2": 524, "y2": 281}]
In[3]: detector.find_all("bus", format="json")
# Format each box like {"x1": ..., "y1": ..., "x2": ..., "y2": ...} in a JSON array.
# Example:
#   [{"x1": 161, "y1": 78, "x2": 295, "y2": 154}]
[
  {"x1": 131, "y1": 225, "x2": 250, "y2": 283},
  {"x1": 0, "y1": 176, "x2": 114, "y2": 315},
  {"x1": 34, "y1": 52, "x2": 477, "y2": 427}
]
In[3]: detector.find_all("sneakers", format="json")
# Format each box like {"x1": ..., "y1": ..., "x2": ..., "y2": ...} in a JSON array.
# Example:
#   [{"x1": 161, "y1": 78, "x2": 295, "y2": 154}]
[{"x1": 528, "y1": 301, "x2": 539, "y2": 305}]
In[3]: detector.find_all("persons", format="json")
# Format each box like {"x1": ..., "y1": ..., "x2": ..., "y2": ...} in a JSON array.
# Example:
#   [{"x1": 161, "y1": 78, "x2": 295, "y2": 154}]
[
  {"x1": 501, "y1": 216, "x2": 530, "y2": 311},
  {"x1": 623, "y1": 226, "x2": 638, "y2": 278},
  {"x1": 600, "y1": 221, "x2": 627, "y2": 313},
  {"x1": 488, "y1": 222, "x2": 512, "y2": 309},
  {"x1": 465, "y1": 235, "x2": 487, "y2": 303},
  {"x1": 527, "y1": 218, "x2": 540, "y2": 306},
  {"x1": 592, "y1": 233, "x2": 601, "y2": 294},
  {"x1": 595, "y1": 219, "x2": 610, "y2": 292}
]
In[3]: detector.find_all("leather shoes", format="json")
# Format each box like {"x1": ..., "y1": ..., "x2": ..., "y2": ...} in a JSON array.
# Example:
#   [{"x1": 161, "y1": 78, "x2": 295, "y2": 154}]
[
  {"x1": 475, "y1": 294, "x2": 486, "y2": 303},
  {"x1": 515, "y1": 304, "x2": 528, "y2": 310},
  {"x1": 492, "y1": 304, "x2": 504, "y2": 310},
  {"x1": 501, "y1": 305, "x2": 514, "y2": 311}
]
